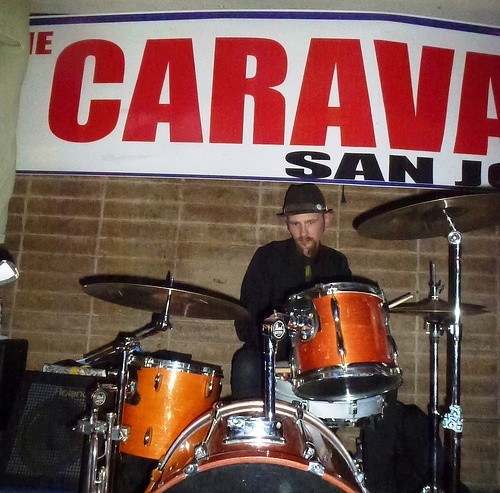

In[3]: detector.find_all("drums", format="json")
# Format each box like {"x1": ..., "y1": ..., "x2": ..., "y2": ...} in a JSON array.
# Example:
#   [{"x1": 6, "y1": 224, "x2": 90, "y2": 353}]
[
  {"x1": 117, "y1": 357, "x2": 222, "y2": 465},
  {"x1": 270, "y1": 361, "x2": 385, "y2": 428},
  {"x1": 147, "y1": 400, "x2": 369, "y2": 493},
  {"x1": 288, "y1": 281, "x2": 403, "y2": 402}
]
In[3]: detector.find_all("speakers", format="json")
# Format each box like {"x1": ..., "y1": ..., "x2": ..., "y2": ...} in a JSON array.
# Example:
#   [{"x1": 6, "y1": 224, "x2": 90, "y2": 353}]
[{"x1": 0, "y1": 369, "x2": 115, "y2": 493}]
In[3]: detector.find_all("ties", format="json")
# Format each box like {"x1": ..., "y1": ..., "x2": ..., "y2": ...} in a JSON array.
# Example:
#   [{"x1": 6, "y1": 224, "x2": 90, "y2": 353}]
[{"x1": 305, "y1": 264, "x2": 312, "y2": 283}]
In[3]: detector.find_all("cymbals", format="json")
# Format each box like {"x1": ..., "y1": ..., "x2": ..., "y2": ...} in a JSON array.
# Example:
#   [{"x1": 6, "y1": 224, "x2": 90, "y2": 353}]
[
  {"x1": 358, "y1": 193, "x2": 500, "y2": 242},
  {"x1": 386, "y1": 297, "x2": 490, "y2": 327}
]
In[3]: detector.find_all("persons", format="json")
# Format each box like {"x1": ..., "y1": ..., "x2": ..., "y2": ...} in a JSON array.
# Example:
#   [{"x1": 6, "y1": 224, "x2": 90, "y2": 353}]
[{"x1": 230, "y1": 183, "x2": 352, "y2": 402}]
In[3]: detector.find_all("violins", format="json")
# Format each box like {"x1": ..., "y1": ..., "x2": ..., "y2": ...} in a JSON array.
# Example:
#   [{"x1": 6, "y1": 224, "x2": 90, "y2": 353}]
[{"x1": 82, "y1": 283, "x2": 250, "y2": 320}]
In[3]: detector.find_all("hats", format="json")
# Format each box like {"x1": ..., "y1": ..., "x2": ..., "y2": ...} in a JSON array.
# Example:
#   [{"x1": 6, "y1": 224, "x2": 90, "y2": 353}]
[{"x1": 276, "y1": 182, "x2": 333, "y2": 216}]
